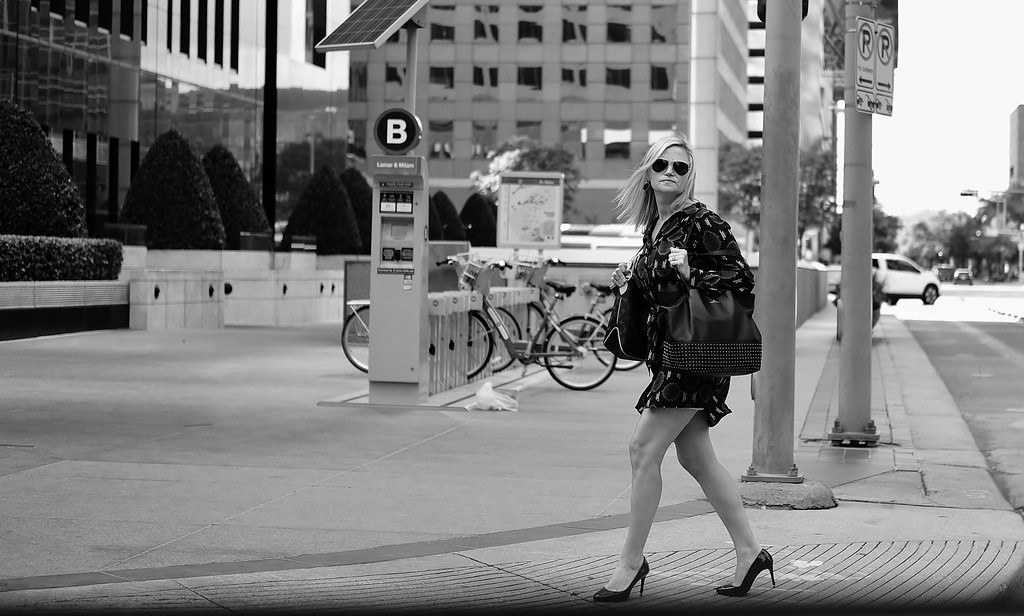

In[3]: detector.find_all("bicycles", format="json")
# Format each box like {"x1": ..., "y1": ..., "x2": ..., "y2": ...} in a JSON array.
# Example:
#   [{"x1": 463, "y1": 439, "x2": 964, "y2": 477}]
[
  {"x1": 491, "y1": 258, "x2": 646, "y2": 371},
  {"x1": 341, "y1": 255, "x2": 494, "y2": 379},
  {"x1": 433, "y1": 256, "x2": 619, "y2": 391}
]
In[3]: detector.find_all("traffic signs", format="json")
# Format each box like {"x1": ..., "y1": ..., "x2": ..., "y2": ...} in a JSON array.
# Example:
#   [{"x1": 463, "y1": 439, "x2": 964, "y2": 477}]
[{"x1": 854, "y1": 15, "x2": 895, "y2": 116}]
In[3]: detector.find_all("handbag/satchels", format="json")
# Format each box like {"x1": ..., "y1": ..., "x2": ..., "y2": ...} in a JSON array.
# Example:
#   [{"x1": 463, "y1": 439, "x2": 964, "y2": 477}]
[
  {"x1": 603, "y1": 282, "x2": 648, "y2": 362},
  {"x1": 653, "y1": 289, "x2": 763, "y2": 376}
]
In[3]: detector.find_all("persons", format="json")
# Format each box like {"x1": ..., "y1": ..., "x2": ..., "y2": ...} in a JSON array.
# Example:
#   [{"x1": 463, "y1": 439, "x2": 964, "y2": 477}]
[{"x1": 588, "y1": 133, "x2": 778, "y2": 602}]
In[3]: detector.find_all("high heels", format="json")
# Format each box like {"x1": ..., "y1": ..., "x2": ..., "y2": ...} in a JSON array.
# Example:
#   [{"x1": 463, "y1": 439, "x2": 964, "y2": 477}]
[
  {"x1": 717, "y1": 549, "x2": 776, "y2": 596},
  {"x1": 593, "y1": 557, "x2": 650, "y2": 602}
]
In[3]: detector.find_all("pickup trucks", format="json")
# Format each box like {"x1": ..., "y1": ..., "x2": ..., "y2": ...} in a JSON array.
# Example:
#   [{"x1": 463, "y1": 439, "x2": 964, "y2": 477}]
[{"x1": 820, "y1": 253, "x2": 941, "y2": 306}]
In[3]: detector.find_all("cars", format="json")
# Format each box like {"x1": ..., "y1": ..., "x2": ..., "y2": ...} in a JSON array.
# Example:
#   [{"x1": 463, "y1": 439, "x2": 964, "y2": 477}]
[{"x1": 952, "y1": 268, "x2": 975, "y2": 286}]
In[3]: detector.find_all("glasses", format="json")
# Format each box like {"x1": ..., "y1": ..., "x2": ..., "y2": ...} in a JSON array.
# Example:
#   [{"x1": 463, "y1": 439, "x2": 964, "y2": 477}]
[{"x1": 653, "y1": 159, "x2": 689, "y2": 176}]
